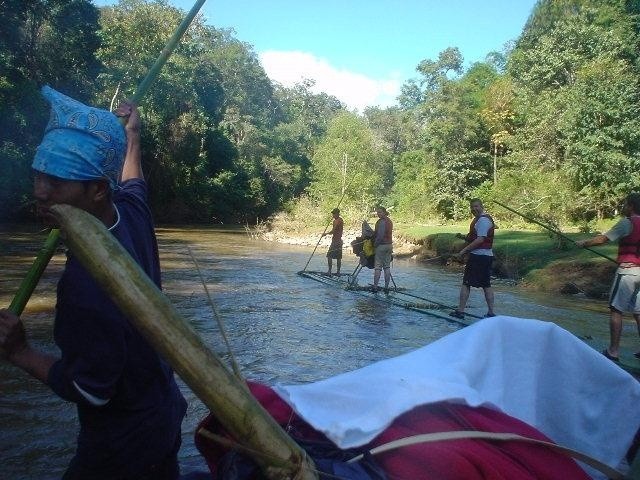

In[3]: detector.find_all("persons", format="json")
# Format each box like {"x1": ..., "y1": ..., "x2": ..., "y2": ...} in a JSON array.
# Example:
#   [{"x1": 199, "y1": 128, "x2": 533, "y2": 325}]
[
  {"x1": 0, "y1": 83, "x2": 189, "y2": 479},
  {"x1": 572, "y1": 193, "x2": 640, "y2": 361},
  {"x1": 369, "y1": 206, "x2": 393, "y2": 294},
  {"x1": 321, "y1": 208, "x2": 345, "y2": 277},
  {"x1": 448, "y1": 198, "x2": 496, "y2": 319}
]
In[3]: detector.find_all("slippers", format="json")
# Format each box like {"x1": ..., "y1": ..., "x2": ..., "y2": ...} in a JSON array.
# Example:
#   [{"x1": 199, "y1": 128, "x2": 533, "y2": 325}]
[{"x1": 600, "y1": 349, "x2": 619, "y2": 361}]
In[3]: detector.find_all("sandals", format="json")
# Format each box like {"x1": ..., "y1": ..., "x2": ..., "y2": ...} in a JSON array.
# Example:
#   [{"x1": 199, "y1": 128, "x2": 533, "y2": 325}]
[{"x1": 450, "y1": 310, "x2": 465, "y2": 319}]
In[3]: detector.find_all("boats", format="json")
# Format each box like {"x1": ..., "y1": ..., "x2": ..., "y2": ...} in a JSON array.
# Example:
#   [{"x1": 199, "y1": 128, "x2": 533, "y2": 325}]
[{"x1": 296, "y1": 270, "x2": 640, "y2": 376}]
